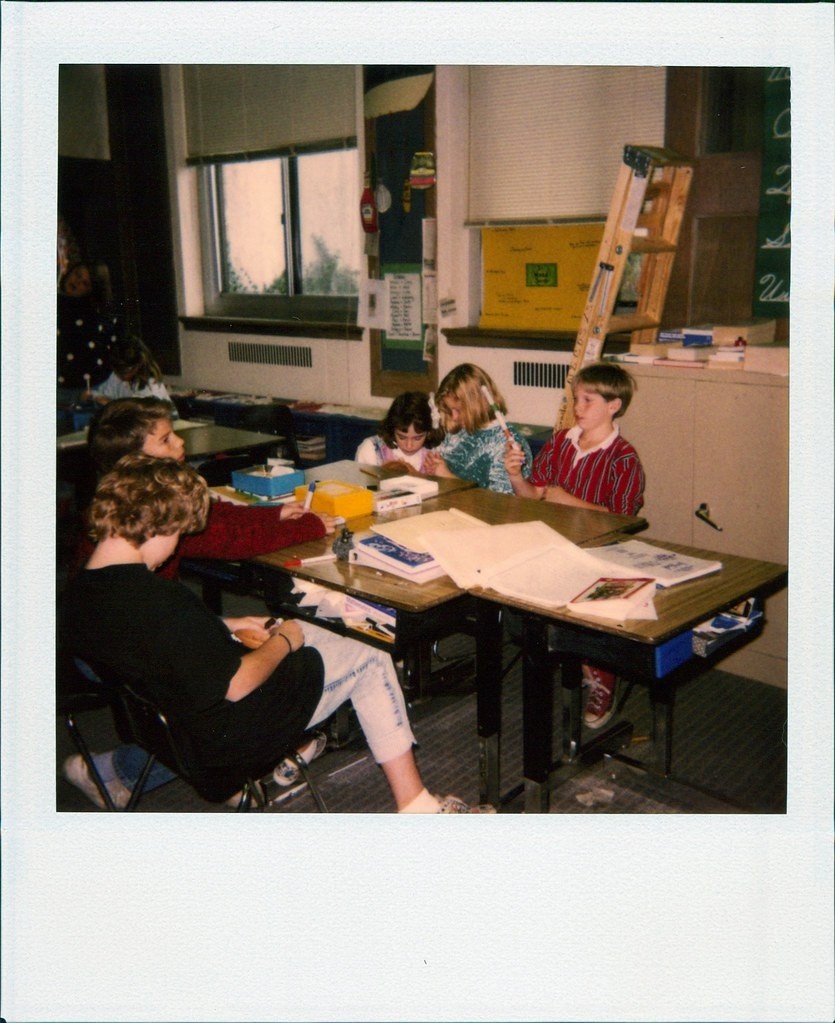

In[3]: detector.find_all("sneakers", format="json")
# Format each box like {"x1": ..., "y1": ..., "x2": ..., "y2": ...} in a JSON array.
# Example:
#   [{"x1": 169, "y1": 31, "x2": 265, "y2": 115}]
[{"x1": 581, "y1": 665, "x2": 621, "y2": 729}]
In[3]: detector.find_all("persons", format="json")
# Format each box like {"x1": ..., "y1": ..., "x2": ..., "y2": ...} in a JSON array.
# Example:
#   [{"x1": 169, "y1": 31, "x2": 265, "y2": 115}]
[
  {"x1": 81, "y1": 334, "x2": 179, "y2": 415},
  {"x1": 72, "y1": 395, "x2": 336, "y2": 581},
  {"x1": 67, "y1": 452, "x2": 496, "y2": 813},
  {"x1": 425, "y1": 363, "x2": 533, "y2": 495},
  {"x1": 355, "y1": 392, "x2": 455, "y2": 478},
  {"x1": 505, "y1": 365, "x2": 646, "y2": 729}
]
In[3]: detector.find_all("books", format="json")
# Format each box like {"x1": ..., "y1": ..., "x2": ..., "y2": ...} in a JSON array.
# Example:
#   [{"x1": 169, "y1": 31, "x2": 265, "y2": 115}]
[
  {"x1": 206, "y1": 484, "x2": 294, "y2": 507},
  {"x1": 348, "y1": 507, "x2": 723, "y2": 621},
  {"x1": 296, "y1": 436, "x2": 327, "y2": 460},
  {"x1": 624, "y1": 318, "x2": 789, "y2": 376}
]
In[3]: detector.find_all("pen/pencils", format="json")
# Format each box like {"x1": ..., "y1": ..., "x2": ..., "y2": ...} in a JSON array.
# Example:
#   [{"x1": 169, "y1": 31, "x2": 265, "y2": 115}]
[
  {"x1": 87, "y1": 377, "x2": 90, "y2": 393},
  {"x1": 283, "y1": 554, "x2": 337, "y2": 566},
  {"x1": 364, "y1": 617, "x2": 394, "y2": 638}
]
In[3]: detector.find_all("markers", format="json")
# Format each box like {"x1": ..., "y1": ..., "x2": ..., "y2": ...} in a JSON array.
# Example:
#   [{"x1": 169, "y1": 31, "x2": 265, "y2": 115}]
[{"x1": 304, "y1": 482, "x2": 317, "y2": 510}]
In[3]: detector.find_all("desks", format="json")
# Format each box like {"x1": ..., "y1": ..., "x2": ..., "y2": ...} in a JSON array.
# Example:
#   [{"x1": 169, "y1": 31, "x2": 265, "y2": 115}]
[{"x1": 55, "y1": 410, "x2": 786, "y2": 814}]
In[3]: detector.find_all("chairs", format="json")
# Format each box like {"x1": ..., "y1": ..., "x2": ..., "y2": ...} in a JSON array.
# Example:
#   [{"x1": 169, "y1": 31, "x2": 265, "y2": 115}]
[{"x1": 60, "y1": 637, "x2": 329, "y2": 812}]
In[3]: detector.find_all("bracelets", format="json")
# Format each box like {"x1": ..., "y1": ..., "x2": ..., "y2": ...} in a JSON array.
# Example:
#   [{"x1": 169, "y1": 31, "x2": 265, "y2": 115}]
[{"x1": 279, "y1": 633, "x2": 292, "y2": 653}]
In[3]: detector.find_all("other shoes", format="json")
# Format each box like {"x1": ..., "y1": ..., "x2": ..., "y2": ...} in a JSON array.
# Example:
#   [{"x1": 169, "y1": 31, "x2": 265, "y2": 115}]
[
  {"x1": 272, "y1": 731, "x2": 327, "y2": 786},
  {"x1": 435, "y1": 795, "x2": 497, "y2": 813},
  {"x1": 65, "y1": 752, "x2": 132, "y2": 810}
]
